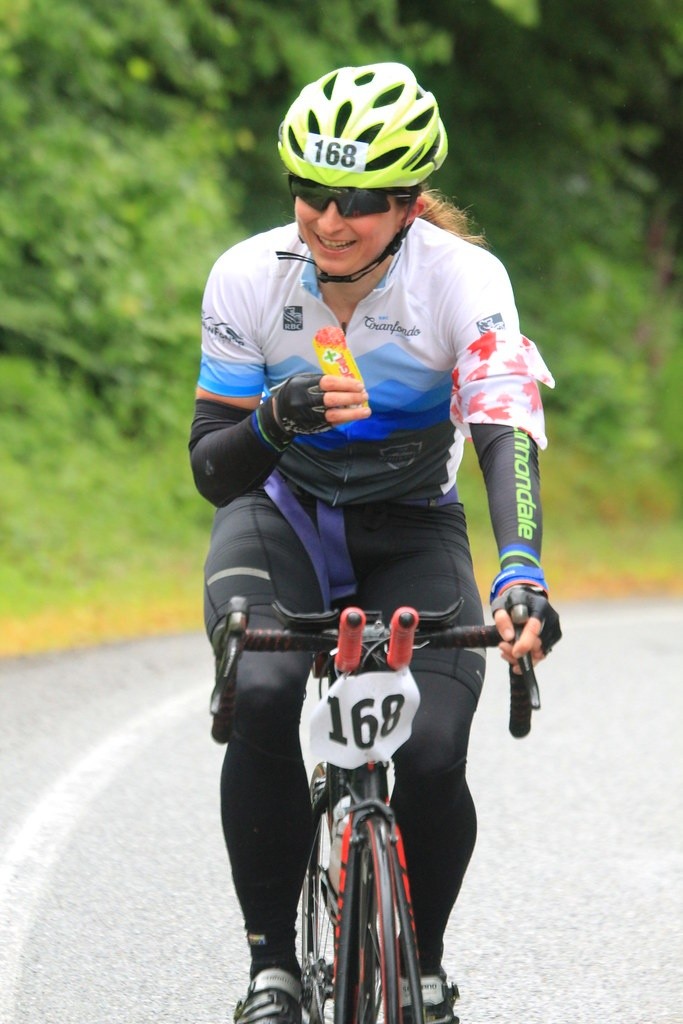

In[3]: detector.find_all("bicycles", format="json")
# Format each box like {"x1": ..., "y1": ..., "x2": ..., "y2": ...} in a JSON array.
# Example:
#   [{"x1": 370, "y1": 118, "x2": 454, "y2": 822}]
[{"x1": 208, "y1": 593, "x2": 540, "y2": 1024}]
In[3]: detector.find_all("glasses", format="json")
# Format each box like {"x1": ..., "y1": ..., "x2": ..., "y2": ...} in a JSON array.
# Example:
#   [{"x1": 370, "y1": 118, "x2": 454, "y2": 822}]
[{"x1": 286, "y1": 171, "x2": 420, "y2": 218}]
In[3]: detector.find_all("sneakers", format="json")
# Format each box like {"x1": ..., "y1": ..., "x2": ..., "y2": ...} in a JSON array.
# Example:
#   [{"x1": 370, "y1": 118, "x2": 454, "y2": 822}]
[
  {"x1": 235, "y1": 968, "x2": 303, "y2": 1024},
  {"x1": 398, "y1": 968, "x2": 461, "y2": 1024}
]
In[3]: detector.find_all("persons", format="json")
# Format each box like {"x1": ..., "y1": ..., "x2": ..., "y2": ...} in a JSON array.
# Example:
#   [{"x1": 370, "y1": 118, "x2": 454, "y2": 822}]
[{"x1": 188, "y1": 55, "x2": 572, "y2": 1024}]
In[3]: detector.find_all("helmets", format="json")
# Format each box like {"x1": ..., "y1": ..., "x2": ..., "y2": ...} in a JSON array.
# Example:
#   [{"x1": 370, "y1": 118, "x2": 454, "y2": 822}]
[{"x1": 277, "y1": 60, "x2": 449, "y2": 190}]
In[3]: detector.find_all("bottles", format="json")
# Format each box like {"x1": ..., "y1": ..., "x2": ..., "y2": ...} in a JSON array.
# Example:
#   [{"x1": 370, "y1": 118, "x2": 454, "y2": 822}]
[{"x1": 328, "y1": 796, "x2": 352, "y2": 895}]
